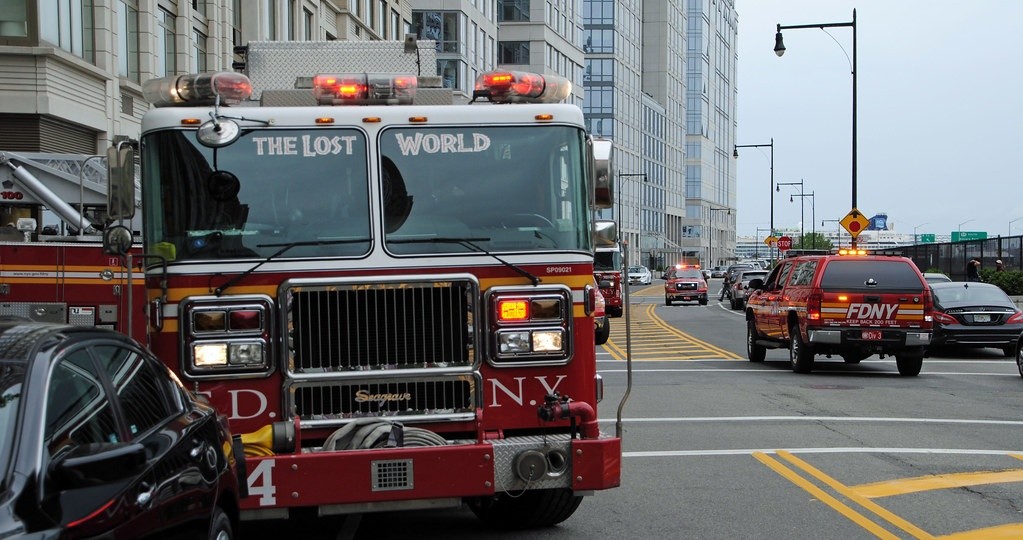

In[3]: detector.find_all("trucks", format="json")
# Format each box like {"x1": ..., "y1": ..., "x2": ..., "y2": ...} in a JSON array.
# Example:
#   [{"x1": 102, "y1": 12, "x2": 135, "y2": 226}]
[{"x1": 590, "y1": 218, "x2": 623, "y2": 317}]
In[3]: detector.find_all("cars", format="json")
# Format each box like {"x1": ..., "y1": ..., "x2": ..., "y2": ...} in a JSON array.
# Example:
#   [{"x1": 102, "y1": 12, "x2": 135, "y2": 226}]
[
  {"x1": 0, "y1": 323, "x2": 240, "y2": 540},
  {"x1": 619, "y1": 265, "x2": 651, "y2": 285},
  {"x1": 920, "y1": 272, "x2": 1023, "y2": 378},
  {"x1": 665, "y1": 260, "x2": 771, "y2": 310}
]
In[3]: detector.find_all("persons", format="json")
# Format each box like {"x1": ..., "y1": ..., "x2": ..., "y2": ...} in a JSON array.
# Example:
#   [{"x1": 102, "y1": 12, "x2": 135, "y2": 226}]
[
  {"x1": 995, "y1": 259, "x2": 1005, "y2": 272},
  {"x1": 967, "y1": 257, "x2": 984, "y2": 282}
]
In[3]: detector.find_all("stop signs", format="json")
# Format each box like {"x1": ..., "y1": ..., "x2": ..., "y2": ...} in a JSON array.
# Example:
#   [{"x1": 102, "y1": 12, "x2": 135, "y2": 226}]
[{"x1": 778, "y1": 237, "x2": 790, "y2": 250}]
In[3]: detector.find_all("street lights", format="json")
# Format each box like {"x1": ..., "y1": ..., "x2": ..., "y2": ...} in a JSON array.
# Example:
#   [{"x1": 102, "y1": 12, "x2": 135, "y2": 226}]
[
  {"x1": 733, "y1": 138, "x2": 774, "y2": 269},
  {"x1": 821, "y1": 219, "x2": 840, "y2": 255},
  {"x1": 710, "y1": 206, "x2": 730, "y2": 268},
  {"x1": 959, "y1": 219, "x2": 976, "y2": 240},
  {"x1": 776, "y1": 179, "x2": 804, "y2": 249},
  {"x1": 619, "y1": 170, "x2": 648, "y2": 250},
  {"x1": 773, "y1": 8, "x2": 857, "y2": 249},
  {"x1": 790, "y1": 191, "x2": 815, "y2": 249},
  {"x1": 914, "y1": 222, "x2": 929, "y2": 244}
]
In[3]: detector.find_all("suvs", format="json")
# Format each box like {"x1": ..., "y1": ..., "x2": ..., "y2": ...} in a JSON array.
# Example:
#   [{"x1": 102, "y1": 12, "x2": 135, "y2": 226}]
[{"x1": 746, "y1": 255, "x2": 934, "y2": 374}]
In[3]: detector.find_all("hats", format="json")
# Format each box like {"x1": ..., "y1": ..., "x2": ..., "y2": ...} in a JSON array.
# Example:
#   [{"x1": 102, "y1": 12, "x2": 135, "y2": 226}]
[{"x1": 995, "y1": 260, "x2": 1002, "y2": 265}]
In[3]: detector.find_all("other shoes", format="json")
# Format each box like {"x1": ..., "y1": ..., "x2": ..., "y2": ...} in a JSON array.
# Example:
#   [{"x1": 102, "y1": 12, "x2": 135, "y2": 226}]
[{"x1": 717, "y1": 298, "x2": 723, "y2": 302}]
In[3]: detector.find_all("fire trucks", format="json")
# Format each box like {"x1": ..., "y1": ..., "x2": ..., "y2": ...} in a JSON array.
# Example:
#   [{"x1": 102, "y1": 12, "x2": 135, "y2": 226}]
[{"x1": 0, "y1": 33, "x2": 633, "y2": 528}]
